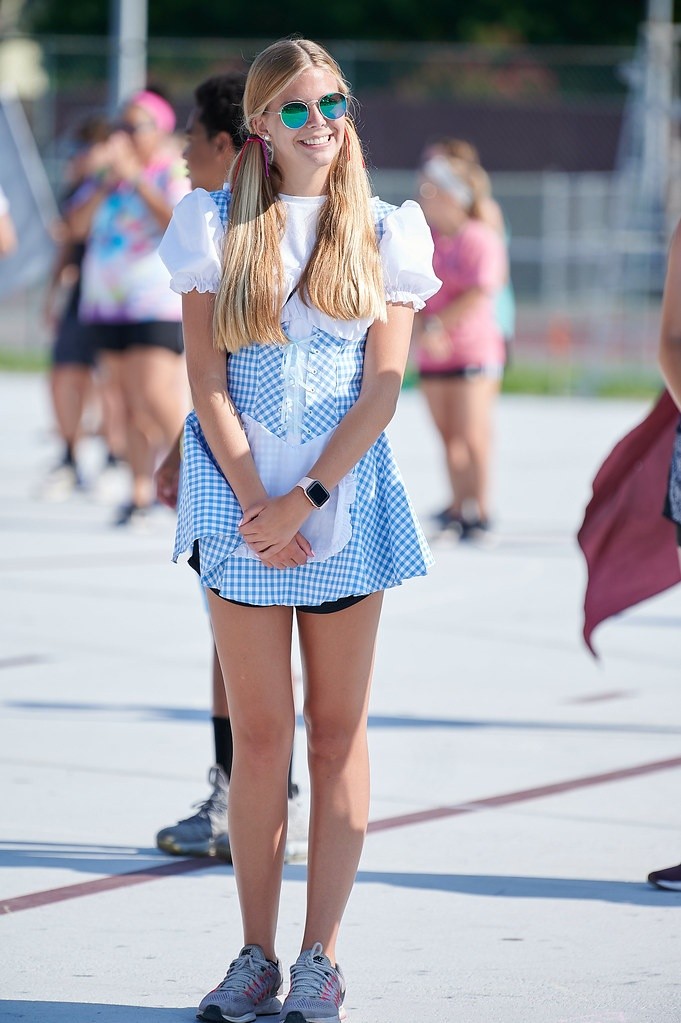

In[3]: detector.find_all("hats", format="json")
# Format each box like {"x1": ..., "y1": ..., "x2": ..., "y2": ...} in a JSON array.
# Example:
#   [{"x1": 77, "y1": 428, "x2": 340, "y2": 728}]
[{"x1": 130, "y1": 87, "x2": 177, "y2": 135}]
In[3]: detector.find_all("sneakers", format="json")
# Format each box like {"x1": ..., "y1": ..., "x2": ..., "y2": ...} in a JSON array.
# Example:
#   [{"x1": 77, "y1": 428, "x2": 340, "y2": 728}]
[
  {"x1": 277, "y1": 942, "x2": 348, "y2": 1023},
  {"x1": 195, "y1": 943, "x2": 284, "y2": 1023},
  {"x1": 154, "y1": 766, "x2": 300, "y2": 859}
]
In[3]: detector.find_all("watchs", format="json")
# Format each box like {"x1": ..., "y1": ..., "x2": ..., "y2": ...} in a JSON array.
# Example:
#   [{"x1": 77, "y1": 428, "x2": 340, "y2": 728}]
[{"x1": 297, "y1": 476, "x2": 331, "y2": 510}]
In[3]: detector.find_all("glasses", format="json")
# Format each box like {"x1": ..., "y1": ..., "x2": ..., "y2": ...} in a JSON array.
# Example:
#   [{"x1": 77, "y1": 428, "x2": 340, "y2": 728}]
[{"x1": 263, "y1": 91, "x2": 349, "y2": 130}]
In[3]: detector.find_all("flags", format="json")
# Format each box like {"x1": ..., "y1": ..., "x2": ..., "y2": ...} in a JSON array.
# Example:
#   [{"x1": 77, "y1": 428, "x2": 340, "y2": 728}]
[{"x1": 577, "y1": 388, "x2": 681, "y2": 659}]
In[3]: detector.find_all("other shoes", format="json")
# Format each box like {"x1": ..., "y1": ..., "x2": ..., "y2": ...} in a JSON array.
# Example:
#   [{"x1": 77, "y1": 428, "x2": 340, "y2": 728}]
[{"x1": 648, "y1": 864, "x2": 681, "y2": 890}]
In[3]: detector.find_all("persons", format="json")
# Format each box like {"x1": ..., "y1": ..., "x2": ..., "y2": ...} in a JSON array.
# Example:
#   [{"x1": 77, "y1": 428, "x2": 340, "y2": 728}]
[
  {"x1": 170, "y1": 39, "x2": 444, "y2": 1023},
  {"x1": 156, "y1": 71, "x2": 250, "y2": 865},
  {"x1": 648, "y1": 219, "x2": 681, "y2": 892},
  {"x1": 1, "y1": 83, "x2": 518, "y2": 539}
]
